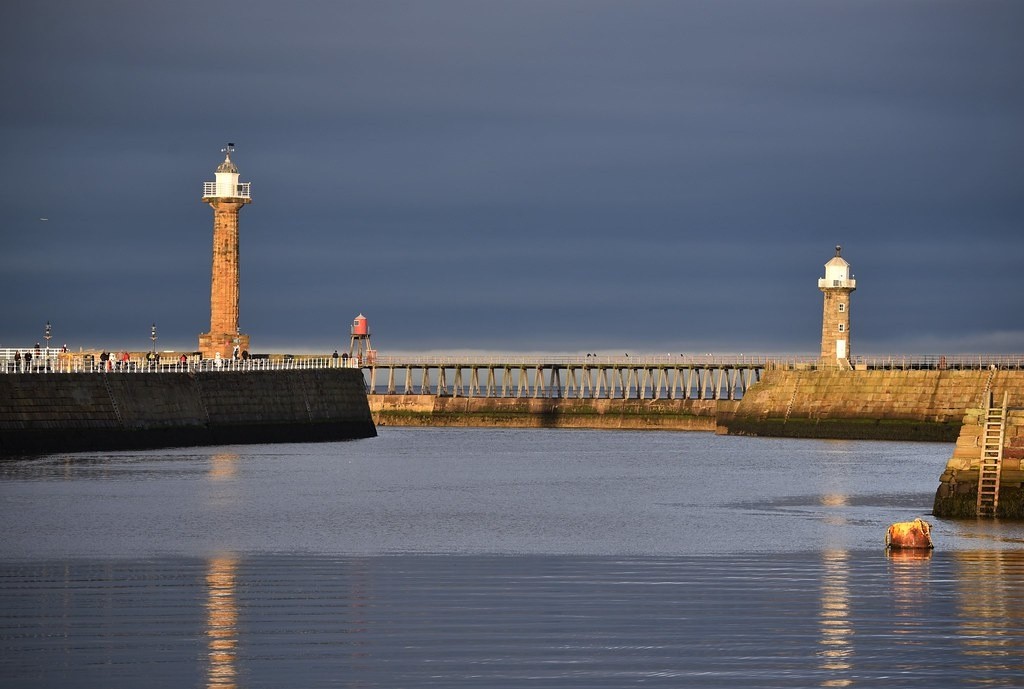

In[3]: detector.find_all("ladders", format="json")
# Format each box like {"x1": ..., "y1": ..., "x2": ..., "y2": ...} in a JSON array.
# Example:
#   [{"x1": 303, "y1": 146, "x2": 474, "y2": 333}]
[{"x1": 976, "y1": 388, "x2": 1008, "y2": 516}]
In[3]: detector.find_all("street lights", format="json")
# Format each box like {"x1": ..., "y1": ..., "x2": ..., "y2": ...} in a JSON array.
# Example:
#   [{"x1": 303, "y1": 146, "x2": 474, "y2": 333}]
[
  {"x1": 43, "y1": 319, "x2": 53, "y2": 348},
  {"x1": 150, "y1": 324, "x2": 159, "y2": 352}
]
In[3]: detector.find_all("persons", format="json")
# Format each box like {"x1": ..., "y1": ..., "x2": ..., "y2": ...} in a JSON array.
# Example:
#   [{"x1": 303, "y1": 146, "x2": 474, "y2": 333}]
[
  {"x1": 100, "y1": 349, "x2": 130, "y2": 369},
  {"x1": 181, "y1": 354, "x2": 187, "y2": 370},
  {"x1": 234, "y1": 348, "x2": 238, "y2": 360},
  {"x1": 146, "y1": 351, "x2": 160, "y2": 370},
  {"x1": 242, "y1": 349, "x2": 248, "y2": 360},
  {"x1": 332, "y1": 350, "x2": 338, "y2": 358},
  {"x1": 342, "y1": 352, "x2": 349, "y2": 360},
  {"x1": 35, "y1": 342, "x2": 40, "y2": 359},
  {"x1": 24, "y1": 350, "x2": 32, "y2": 372},
  {"x1": 61, "y1": 343, "x2": 68, "y2": 352},
  {"x1": 15, "y1": 350, "x2": 21, "y2": 371}
]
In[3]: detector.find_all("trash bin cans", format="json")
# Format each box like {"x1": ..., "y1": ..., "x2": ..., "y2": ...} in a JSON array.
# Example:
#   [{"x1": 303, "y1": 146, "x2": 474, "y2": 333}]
[{"x1": 82, "y1": 354, "x2": 95, "y2": 372}]
